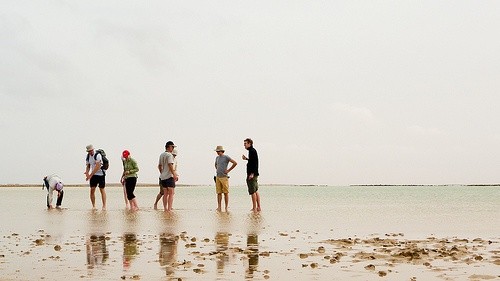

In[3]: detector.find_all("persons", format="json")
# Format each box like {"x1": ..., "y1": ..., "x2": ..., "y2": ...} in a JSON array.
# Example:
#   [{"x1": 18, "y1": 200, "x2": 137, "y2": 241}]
[
  {"x1": 158, "y1": 141, "x2": 177, "y2": 212},
  {"x1": 159, "y1": 240, "x2": 178, "y2": 274},
  {"x1": 215, "y1": 233, "x2": 229, "y2": 268},
  {"x1": 120, "y1": 150, "x2": 140, "y2": 211},
  {"x1": 154, "y1": 149, "x2": 179, "y2": 210},
  {"x1": 242, "y1": 138, "x2": 262, "y2": 212},
  {"x1": 43, "y1": 174, "x2": 65, "y2": 210},
  {"x1": 84, "y1": 144, "x2": 107, "y2": 210},
  {"x1": 86, "y1": 235, "x2": 109, "y2": 266},
  {"x1": 123, "y1": 232, "x2": 139, "y2": 266},
  {"x1": 214, "y1": 146, "x2": 238, "y2": 211},
  {"x1": 248, "y1": 235, "x2": 260, "y2": 273}
]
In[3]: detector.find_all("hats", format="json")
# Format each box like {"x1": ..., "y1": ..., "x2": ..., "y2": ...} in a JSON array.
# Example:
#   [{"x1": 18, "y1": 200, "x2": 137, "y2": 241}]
[
  {"x1": 85, "y1": 144, "x2": 95, "y2": 152},
  {"x1": 56, "y1": 180, "x2": 64, "y2": 190},
  {"x1": 171, "y1": 149, "x2": 178, "y2": 155},
  {"x1": 122, "y1": 150, "x2": 131, "y2": 158},
  {"x1": 165, "y1": 141, "x2": 177, "y2": 147},
  {"x1": 214, "y1": 146, "x2": 225, "y2": 151}
]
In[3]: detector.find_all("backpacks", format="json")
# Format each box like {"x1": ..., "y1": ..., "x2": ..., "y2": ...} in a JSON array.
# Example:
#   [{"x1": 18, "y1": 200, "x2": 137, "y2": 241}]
[{"x1": 96, "y1": 148, "x2": 109, "y2": 170}]
